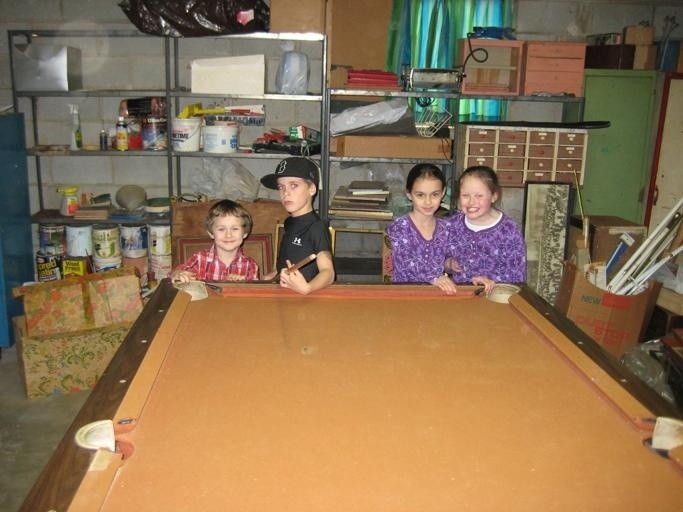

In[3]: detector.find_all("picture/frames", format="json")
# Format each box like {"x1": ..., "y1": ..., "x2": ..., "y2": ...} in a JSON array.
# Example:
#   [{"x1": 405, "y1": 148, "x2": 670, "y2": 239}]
[
  {"x1": 521, "y1": 180, "x2": 573, "y2": 307},
  {"x1": 272, "y1": 224, "x2": 336, "y2": 272},
  {"x1": 176, "y1": 233, "x2": 273, "y2": 275}
]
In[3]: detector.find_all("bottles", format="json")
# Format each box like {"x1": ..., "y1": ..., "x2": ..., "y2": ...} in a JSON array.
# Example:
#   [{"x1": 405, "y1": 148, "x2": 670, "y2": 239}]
[
  {"x1": 99, "y1": 129, "x2": 107, "y2": 152},
  {"x1": 115, "y1": 115, "x2": 128, "y2": 152}
]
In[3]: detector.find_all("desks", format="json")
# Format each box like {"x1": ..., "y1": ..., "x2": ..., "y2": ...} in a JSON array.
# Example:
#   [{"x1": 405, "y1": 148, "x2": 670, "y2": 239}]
[{"x1": 19, "y1": 278, "x2": 683, "y2": 512}]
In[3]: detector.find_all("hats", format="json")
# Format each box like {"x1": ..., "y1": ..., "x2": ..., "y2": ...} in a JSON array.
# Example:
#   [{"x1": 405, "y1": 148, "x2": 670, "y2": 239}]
[{"x1": 259, "y1": 157, "x2": 320, "y2": 191}]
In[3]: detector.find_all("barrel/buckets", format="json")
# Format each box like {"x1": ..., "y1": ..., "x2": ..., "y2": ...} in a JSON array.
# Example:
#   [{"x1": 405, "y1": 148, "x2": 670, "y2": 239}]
[{"x1": 172, "y1": 118, "x2": 202, "y2": 152}]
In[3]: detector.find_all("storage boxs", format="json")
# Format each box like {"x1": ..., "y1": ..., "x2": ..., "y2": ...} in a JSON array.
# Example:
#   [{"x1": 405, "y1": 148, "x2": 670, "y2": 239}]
[
  {"x1": 23, "y1": 265, "x2": 145, "y2": 338},
  {"x1": 568, "y1": 261, "x2": 663, "y2": 357},
  {"x1": 192, "y1": 53, "x2": 265, "y2": 95},
  {"x1": 329, "y1": 136, "x2": 338, "y2": 154},
  {"x1": 336, "y1": 134, "x2": 452, "y2": 160},
  {"x1": 12, "y1": 298, "x2": 151, "y2": 398}
]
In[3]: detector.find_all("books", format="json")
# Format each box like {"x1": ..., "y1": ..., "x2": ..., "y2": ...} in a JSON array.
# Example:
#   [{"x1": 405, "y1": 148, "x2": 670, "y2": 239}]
[
  {"x1": 346, "y1": 69, "x2": 400, "y2": 89},
  {"x1": 327, "y1": 178, "x2": 395, "y2": 220}
]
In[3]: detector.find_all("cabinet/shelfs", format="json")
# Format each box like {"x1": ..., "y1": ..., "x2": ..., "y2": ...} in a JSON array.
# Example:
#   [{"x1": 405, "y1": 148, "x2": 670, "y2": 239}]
[
  {"x1": 458, "y1": 38, "x2": 524, "y2": 96},
  {"x1": 456, "y1": 124, "x2": 588, "y2": 188},
  {"x1": 0, "y1": 114, "x2": 34, "y2": 347},
  {"x1": 327, "y1": 88, "x2": 586, "y2": 280},
  {"x1": 7, "y1": 29, "x2": 327, "y2": 261},
  {"x1": 520, "y1": 41, "x2": 588, "y2": 97}
]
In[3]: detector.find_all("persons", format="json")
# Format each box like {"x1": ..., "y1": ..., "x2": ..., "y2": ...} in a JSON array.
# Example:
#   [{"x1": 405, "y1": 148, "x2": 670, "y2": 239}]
[
  {"x1": 385, "y1": 161, "x2": 498, "y2": 296},
  {"x1": 443, "y1": 165, "x2": 528, "y2": 283},
  {"x1": 259, "y1": 156, "x2": 336, "y2": 296},
  {"x1": 167, "y1": 198, "x2": 277, "y2": 285}
]
